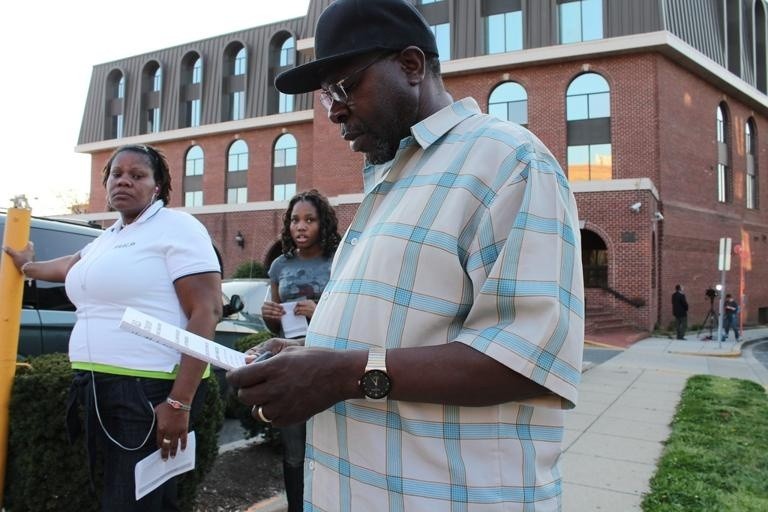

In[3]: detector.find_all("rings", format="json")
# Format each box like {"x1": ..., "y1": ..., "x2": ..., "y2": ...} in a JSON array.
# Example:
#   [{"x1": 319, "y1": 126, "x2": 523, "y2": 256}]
[
  {"x1": 257, "y1": 408, "x2": 273, "y2": 423},
  {"x1": 163, "y1": 438, "x2": 170, "y2": 443}
]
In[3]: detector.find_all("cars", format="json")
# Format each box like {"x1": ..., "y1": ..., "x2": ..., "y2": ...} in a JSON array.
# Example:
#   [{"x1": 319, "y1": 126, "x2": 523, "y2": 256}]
[
  {"x1": 219, "y1": 277, "x2": 275, "y2": 319},
  {"x1": 0, "y1": 211, "x2": 276, "y2": 413}
]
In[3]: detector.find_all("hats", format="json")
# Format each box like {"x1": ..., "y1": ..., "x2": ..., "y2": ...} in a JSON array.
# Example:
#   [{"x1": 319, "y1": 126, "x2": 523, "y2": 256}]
[{"x1": 274, "y1": 1, "x2": 439, "y2": 94}]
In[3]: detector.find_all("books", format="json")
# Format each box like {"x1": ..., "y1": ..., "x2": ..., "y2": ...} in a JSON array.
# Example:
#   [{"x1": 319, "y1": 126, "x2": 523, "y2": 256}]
[
  {"x1": 134, "y1": 430, "x2": 197, "y2": 502},
  {"x1": 116, "y1": 304, "x2": 248, "y2": 376},
  {"x1": 278, "y1": 300, "x2": 310, "y2": 340}
]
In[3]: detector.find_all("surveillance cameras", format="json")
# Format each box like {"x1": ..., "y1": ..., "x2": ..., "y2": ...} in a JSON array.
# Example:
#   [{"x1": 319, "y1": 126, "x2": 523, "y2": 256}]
[
  {"x1": 630, "y1": 203, "x2": 642, "y2": 211},
  {"x1": 655, "y1": 212, "x2": 665, "y2": 220}
]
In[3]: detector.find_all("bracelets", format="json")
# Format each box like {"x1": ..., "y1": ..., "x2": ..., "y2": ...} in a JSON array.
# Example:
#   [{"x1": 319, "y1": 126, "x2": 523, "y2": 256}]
[{"x1": 22, "y1": 261, "x2": 35, "y2": 280}]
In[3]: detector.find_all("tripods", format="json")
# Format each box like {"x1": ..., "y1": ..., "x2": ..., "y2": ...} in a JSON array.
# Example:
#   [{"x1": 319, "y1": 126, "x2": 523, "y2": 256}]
[{"x1": 697, "y1": 298, "x2": 718, "y2": 339}]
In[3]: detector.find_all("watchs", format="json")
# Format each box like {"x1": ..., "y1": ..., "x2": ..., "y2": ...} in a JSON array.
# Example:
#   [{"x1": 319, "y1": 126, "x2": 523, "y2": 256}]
[
  {"x1": 166, "y1": 396, "x2": 193, "y2": 412},
  {"x1": 358, "y1": 346, "x2": 392, "y2": 403}
]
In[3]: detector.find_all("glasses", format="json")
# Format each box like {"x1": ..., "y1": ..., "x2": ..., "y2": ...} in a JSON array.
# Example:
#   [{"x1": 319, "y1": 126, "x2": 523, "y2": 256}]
[{"x1": 320, "y1": 53, "x2": 387, "y2": 111}]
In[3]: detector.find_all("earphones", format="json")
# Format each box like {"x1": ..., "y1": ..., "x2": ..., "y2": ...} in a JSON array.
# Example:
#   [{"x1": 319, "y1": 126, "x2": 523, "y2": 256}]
[
  {"x1": 156, "y1": 187, "x2": 159, "y2": 191},
  {"x1": 124, "y1": 228, "x2": 127, "y2": 232}
]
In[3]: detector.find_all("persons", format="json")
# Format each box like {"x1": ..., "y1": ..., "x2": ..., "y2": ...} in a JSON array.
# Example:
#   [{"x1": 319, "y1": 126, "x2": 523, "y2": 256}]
[
  {"x1": 4, "y1": 142, "x2": 224, "y2": 511},
  {"x1": 723, "y1": 294, "x2": 743, "y2": 342},
  {"x1": 261, "y1": 189, "x2": 343, "y2": 510},
  {"x1": 226, "y1": 1, "x2": 588, "y2": 510},
  {"x1": 672, "y1": 284, "x2": 689, "y2": 340}
]
